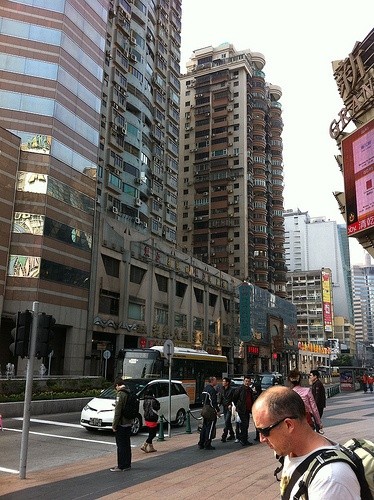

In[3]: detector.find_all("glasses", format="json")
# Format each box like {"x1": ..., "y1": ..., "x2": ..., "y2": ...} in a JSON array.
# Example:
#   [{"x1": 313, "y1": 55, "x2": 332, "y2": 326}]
[{"x1": 255, "y1": 415, "x2": 298, "y2": 437}]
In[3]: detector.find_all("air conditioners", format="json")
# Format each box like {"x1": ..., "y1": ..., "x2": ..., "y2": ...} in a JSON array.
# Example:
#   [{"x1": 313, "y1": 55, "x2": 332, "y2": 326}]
[{"x1": 102, "y1": 0, "x2": 238, "y2": 268}]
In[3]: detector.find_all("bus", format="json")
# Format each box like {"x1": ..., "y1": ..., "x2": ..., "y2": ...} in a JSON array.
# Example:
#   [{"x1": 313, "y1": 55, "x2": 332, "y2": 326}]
[{"x1": 113, "y1": 344, "x2": 229, "y2": 408}]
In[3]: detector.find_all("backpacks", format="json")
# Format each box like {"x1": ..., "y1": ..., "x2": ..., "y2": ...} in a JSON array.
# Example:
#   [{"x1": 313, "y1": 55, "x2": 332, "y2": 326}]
[
  {"x1": 292, "y1": 432, "x2": 374, "y2": 500},
  {"x1": 118, "y1": 389, "x2": 140, "y2": 420}
]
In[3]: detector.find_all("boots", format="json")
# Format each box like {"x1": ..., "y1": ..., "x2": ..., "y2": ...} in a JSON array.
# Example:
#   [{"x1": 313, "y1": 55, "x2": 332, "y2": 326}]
[
  {"x1": 140, "y1": 440, "x2": 149, "y2": 453},
  {"x1": 148, "y1": 444, "x2": 157, "y2": 452}
]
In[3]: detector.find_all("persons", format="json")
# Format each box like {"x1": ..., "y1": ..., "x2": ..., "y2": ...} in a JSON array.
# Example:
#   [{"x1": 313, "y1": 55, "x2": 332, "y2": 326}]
[
  {"x1": 361, "y1": 372, "x2": 374, "y2": 394},
  {"x1": 250, "y1": 384, "x2": 361, "y2": 500},
  {"x1": 140, "y1": 390, "x2": 160, "y2": 452},
  {"x1": 198, "y1": 375, "x2": 263, "y2": 450},
  {"x1": 273, "y1": 369, "x2": 326, "y2": 481},
  {"x1": 110, "y1": 377, "x2": 136, "y2": 471}
]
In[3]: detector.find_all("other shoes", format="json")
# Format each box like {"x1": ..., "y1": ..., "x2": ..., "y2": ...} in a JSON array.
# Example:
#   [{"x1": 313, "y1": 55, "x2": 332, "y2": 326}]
[
  {"x1": 239, "y1": 439, "x2": 253, "y2": 445},
  {"x1": 227, "y1": 435, "x2": 235, "y2": 440},
  {"x1": 235, "y1": 438, "x2": 239, "y2": 443},
  {"x1": 200, "y1": 445, "x2": 205, "y2": 449},
  {"x1": 254, "y1": 438, "x2": 260, "y2": 441},
  {"x1": 221, "y1": 439, "x2": 226, "y2": 442},
  {"x1": 198, "y1": 442, "x2": 201, "y2": 446},
  {"x1": 124, "y1": 467, "x2": 131, "y2": 471},
  {"x1": 205, "y1": 446, "x2": 216, "y2": 450},
  {"x1": 109, "y1": 467, "x2": 122, "y2": 472}
]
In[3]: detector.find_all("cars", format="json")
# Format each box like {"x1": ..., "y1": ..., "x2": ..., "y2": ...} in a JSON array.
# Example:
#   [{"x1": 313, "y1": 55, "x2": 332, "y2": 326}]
[
  {"x1": 78, "y1": 378, "x2": 190, "y2": 437},
  {"x1": 228, "y1": 371, "x2": 284, "y2": 403}
]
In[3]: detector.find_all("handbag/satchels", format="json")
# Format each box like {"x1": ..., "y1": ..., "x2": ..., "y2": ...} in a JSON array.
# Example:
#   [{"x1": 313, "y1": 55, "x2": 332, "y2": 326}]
[
  {"x1": 201, "y1": 405, "x2": 214, "y2": 418},
  {"x1": 148, "y1": 399, "x2": 159, "y2": 420},
  {"x1": 230, "y1": 402, "x2": 241, "y2": 423}
]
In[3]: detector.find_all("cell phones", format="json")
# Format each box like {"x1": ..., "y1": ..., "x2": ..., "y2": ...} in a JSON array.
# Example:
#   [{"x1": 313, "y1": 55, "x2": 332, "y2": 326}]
[{"x1": 112, "y1": 404, "x2": 116, "y2": 406}]
